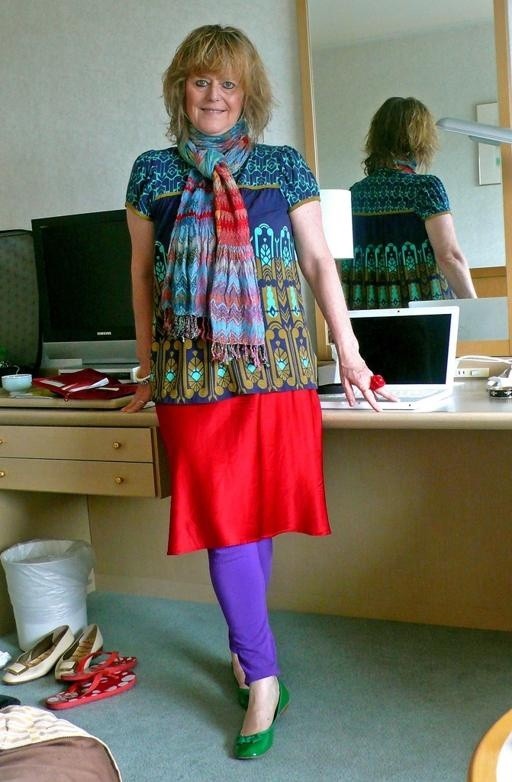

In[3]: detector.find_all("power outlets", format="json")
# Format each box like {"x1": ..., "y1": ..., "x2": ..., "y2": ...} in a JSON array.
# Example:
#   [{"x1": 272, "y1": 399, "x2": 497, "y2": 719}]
[{"x1": 455, "y1": 367, "x2": 490, "y2": 378}]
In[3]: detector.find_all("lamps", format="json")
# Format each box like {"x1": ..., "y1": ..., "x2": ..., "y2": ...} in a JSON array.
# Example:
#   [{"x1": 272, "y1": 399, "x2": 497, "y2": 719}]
[
  {"x1": 312, "y1": 187, "x2": 357, "y2": 399},
  {"x1": 434, "y1": 118, "x2": 512, "y2": 147}
]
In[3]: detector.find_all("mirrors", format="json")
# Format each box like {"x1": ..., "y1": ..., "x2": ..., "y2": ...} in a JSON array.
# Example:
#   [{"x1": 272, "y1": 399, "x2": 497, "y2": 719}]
[{"x1": 293, "y1": 0, "x2": 512, "y2": 362}]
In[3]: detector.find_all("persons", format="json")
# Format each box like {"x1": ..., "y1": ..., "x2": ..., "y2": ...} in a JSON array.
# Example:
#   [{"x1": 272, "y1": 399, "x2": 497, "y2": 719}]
[
  {"x1": 119, "y1": 23, "x2": 399, "y2": 760},
  {"x1": 337, "y1": 96, "x2": 486, "y2": 309}
]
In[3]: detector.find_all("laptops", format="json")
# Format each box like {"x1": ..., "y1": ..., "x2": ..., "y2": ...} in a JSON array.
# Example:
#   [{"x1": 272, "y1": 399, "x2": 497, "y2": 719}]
[
  {"x1": 408, "y1": 297, "x2": 507, "y2": 343},
  {"x1": 315, "y1": 305, "x2": 459, "y2": 410}
]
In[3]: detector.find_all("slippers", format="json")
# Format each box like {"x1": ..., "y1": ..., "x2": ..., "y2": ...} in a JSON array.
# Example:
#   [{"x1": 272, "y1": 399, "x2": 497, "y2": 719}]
[
  {"x1": 61, "y1": 651, "x2": 136, "y2": 682},
  {"x1": 46, "y1": 671, "x2": 136, "y2": 710}
]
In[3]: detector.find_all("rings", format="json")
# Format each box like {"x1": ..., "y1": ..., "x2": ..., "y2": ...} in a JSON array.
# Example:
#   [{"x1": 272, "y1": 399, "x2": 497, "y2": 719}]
[
  {"x1": 138, "y1": 398, "x2": 145, "y2": 404},
  {"x1": 369, "y1": 373, "x2": 387, "y2": 392}
]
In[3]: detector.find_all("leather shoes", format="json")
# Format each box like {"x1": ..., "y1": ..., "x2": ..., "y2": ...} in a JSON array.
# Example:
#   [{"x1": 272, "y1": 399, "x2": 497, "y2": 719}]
[
  {"x1": 55, "y1": 624, "x2": 103, "y2": 681},
  {"x1": 227, "y1": 662, "x2": 249, "y2": 710},
  {"x1": 233, "y1": 678, "x2": 289, "y2": 759},
  {"x1": 3, "y1": 625, "x2": 75, "y2": 685}
]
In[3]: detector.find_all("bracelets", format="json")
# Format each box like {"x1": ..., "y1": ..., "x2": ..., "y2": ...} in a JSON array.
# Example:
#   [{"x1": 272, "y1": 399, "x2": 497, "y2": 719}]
[{"x1": 135, "y1": 374, "x2": 150, "y2": 384}]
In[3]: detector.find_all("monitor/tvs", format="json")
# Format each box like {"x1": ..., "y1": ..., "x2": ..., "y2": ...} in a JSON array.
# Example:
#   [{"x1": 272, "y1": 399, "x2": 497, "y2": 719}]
[{"x1": 32, "y1": 209, "x2": 156, "y2": 368}]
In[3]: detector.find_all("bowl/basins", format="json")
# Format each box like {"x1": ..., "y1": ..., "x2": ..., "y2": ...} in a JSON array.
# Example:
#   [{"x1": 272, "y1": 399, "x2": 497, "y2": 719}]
[{"x1": 0, "y1": 373, "x2": 33, "y2": 396}]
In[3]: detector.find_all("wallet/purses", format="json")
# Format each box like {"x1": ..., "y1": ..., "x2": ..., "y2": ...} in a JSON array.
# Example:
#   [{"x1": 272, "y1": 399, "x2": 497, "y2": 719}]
[{"x1": 32, "y1": 367, "x2": 136, "y2": 401}]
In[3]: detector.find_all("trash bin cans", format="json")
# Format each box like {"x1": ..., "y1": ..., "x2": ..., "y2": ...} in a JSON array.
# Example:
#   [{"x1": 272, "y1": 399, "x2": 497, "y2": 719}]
[{"x1": 1, "y1": 539, "x2": 94, "y2": 650}]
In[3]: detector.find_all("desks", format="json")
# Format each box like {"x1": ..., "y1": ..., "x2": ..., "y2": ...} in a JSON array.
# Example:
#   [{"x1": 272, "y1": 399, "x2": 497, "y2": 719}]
[{"x1": 0, "y1": 382, "x2": 512, "y2": 637}]
